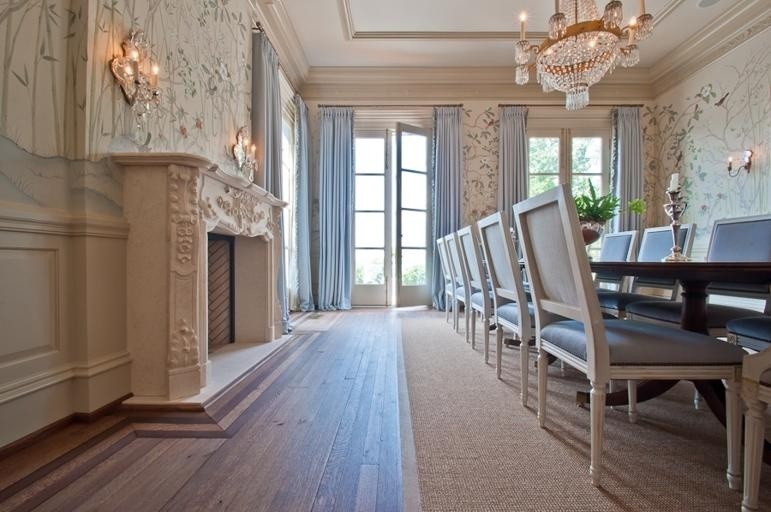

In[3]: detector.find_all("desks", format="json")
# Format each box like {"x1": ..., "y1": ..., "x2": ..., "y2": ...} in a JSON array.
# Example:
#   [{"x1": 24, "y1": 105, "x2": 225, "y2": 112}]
[{"x1": 488, "y1": 261, "x2": 770, "y2": 464}]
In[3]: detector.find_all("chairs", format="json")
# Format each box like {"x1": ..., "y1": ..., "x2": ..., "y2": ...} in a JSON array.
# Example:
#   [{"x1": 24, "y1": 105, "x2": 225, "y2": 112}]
[
  {"x1": 593, "y1": 230, "x2": 640, "y2": 293},
  {"x1": 621, "y1": 213, "x2": 771, "y2": 411},
  {"x1": 512, "y1": 184, "x2": 749, "y2": 487},
  {"x1": 596, "y1": 222, "x2": 697, "y2": 318},
  {"x1": 435, "y1": 211, "x2": 619, "y2": 408}
]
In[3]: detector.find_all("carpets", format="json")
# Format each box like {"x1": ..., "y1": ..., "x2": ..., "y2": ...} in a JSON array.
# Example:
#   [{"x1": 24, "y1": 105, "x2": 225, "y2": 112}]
[{"x1": 398, "y1": 305, "x2": 770, "y2": 512}]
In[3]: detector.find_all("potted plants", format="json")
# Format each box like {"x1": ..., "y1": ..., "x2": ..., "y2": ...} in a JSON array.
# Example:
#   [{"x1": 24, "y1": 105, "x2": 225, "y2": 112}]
[{"x1": 572, "y1": 178, "x2": 648, "y2": 259}]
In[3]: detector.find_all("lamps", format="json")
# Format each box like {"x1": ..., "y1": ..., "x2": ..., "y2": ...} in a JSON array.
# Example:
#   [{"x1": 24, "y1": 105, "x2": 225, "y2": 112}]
[
  {"x1": 113, "y1": 31, "x2": 167, "y2": 116},
  {"x1": 727, "y1": 150, "x2": 753, "y2": 177},
  {"x1": 514, "y1": 0, "x2": 653, "y2": 110},
  {"x1": 233, "y1": 125, "x2": 259, "y2": 178}
]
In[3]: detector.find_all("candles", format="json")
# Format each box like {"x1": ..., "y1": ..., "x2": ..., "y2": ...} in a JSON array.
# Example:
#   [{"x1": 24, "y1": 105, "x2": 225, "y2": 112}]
[{"x1": 669, "y1": 173, "x2": 679, "y2": 191}]
